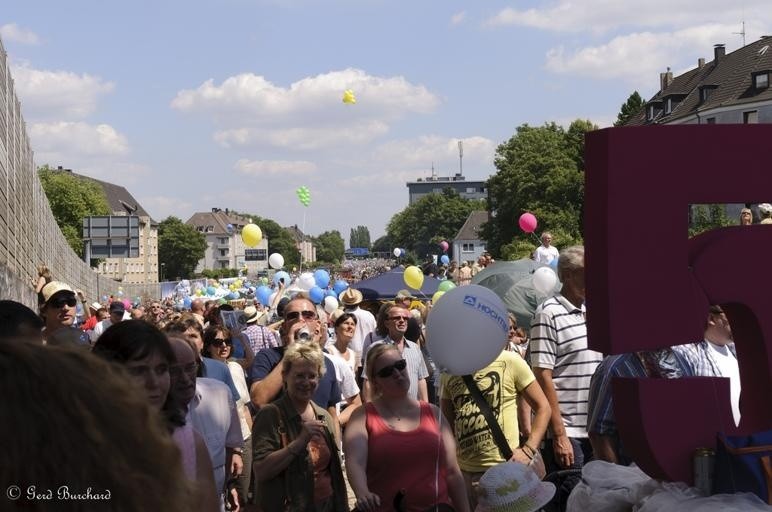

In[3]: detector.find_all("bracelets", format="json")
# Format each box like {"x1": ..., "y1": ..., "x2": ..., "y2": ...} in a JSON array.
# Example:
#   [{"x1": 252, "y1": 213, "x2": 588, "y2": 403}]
[
  {"x1": 522, "y1": 443, "x2": 537, "y2": 459},
  {"x1": 287, "y1": 444, "x2": 301, "y2": 458}
]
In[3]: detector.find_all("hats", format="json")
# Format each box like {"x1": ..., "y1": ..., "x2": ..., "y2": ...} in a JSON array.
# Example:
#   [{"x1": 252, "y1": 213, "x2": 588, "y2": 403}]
[
  {"x1": 109, "y1": 301, "x2": 125, "y2": 314},
  {"x1": 395, "y1": 289, "x2": 417, "y2": 299},
  {"x1": 338, "y1": 287, "x2": 363, "y2": 305},
  {"x1": 237, "y1": 306, "x2": 262, "y2": 324},
  {"x1": 173, "y1": 311, "x2": 181, "y2": 318},
  {"x1": 474, "y1": 461, "x2": 557, "y2": 512},
  {"x1": 90, "y1": 302, "x2": 102, "y2": 311},
  {"x1": 37, "y1": 280, "x2": 76, "y2": 304}
]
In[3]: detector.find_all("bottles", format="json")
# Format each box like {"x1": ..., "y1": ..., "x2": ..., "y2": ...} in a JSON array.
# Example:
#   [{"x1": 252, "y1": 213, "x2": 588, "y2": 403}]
[{"x1": 693, "y1": 448, "x2": 717, "y2": 498}]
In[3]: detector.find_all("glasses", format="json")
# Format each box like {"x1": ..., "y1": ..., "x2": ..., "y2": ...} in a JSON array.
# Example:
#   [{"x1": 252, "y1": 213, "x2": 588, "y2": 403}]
[
  {"x1": 509, "y1": 325, "x2": 518, "y2": 330},
  {"x1": 386, "y1": 316, "x2": 409, "y2": 320},
  {"x1": 52, "y1": 297, "x2": 77, "y2": 308},
  {"x1": 286, "y1": 310, "x2": 315, "y2": 320},
  {"x1": 169, "y1": 363, "x2": 201, "y2": 376},
  {"x1": 373, "y1": 358, "x2": 407, "y2": 378},
  {"x1": 208, "y1": 337, "x2": 232, "y2": 346},
  {"x1": 151, "y1": 305, "x2": 160, "y2": 308}
]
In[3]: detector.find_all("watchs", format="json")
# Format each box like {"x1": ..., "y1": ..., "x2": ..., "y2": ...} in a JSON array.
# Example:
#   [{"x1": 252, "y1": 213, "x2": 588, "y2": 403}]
[{"x1": 232, "y1": 450, "x2": 243, "y2": 457}]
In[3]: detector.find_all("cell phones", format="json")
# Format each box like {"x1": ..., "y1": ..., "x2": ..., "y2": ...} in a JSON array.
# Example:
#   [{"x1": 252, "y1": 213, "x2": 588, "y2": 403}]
[{"x1": 280, "y1": 277, "x2": 285, "y2": 284}]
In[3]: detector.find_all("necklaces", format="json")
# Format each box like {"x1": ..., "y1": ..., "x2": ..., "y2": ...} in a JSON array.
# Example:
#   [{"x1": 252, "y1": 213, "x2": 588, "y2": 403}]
[
  {"x1": 294, "y1": 408, "x2": 315, "y2": 423},
  {"x1": 381, "y1": 396, "x2": 410, "y2": 421}
]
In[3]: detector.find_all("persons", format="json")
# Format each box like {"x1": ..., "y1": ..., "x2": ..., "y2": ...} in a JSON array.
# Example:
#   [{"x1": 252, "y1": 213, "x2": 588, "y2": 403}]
[
  {"x1": 242, "y1": 306, "x2": 278, "y2": 356},
  {"x1": 425, "y1": 263, "x2": 447, "y2": 281},
  {"x1": 531, "y1": 245, "x2": 604, "y2": 512},
  {"x1": 34, "y1": 264, "x2": 53, "y2": 309},
  {"x1": 202, "y1": 325, "x2": 252, "y2": 512},
  {"x1": 255, "y1": 278, "x2": 286, "y2": 326},
  {"x1": 325, "y1": 313, "x2": 360, "y2": 379},
  {"x1": 171, "y1": 319, "x2": 242, "y2": 401},
  {"x1": 471, "y1": 257, "x2": 486, "y2": 277},
  {"x1": 586, "y1": 344, "x2": 707, "y2": 465},
  {"x1": 484, "y1": 253, "x2": 491, "y2": 267},
  {"x1": 361, "y1": 307, "x2": 429, "y2": 402},
  {"x1": 254, "y1": 296, "x2": 342, "y2": 466},
  {"x1": 330, "y1": 259, "x2": 397, "y2": 285},
  {"x1": 163, "y1": 332, "x2": 244, "y2": 512},
  {"x1": 673, "y1": 305, "x2": 741, "y2": 429},
  {"x1": 446, "y1": 259, "x2": 459, "y2": 283},
  {"x1": 1, "y1": 300, "x2": 41, "y2": 354},
  {"x1": 342, "y1": 344, "x2": 471, "y2": 512},
  {"x1": 459, "y1": 261, "x2": 472, "y2": 286},
  {"x1": 410, "y1": 308, "x2": 433, "y2": 381},
  {"x1": 756, "y1": 203, "x2": 772, "y2": 224},
  {"x1": 395, "y1": 289, "x2": 426, "y2": 314},
  {"x1": 339, "y1": 287, "x2": 377, "y2": 381},
  {"x1": 438, "y1": 348, "x2": 551, "y2": 512},
  {"x1": 740, "y1": 208, "x2": 752, "y2": 225},
  {"x1": 503, "y1": 314, "x2": 521, "y2": 352},
  {"x1": 77, "y1": 287, "x2": 134, "y2": 319},
  {"x1": 516, "y1": 327, "x2": 530, "y2": 358},
  {"x1": 0, "y1": 340, "x2": 194, "y2": 512},
  {"x1": 536, "y1": 232, "x2": 558, "y2": 264},
  {"x1": 92, "y1": 320, "x2": 220, "y2": 512},
  {"x1": 37, "y1": 281, "x2": 76, "y2": 348},
  {"x1": 362, "y1": 303, "x2": 406, "y2": 401},
  {"x1": 409, "y1": 300, "x2": 426, "y2": 344},
  {"x1": 134, "y1": 294, "x2": 245, "y2": 320},
  {"x1": 251, "y1": 343, "x2": 348, "y2": 512}
]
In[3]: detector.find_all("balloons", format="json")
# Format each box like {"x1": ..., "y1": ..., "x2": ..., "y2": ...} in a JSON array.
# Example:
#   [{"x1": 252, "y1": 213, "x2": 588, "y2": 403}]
[
  {"x1": 269, "y1": 253, "x2": 285, "y2": 269},
  {"x1": 256, "y1": 284, "x2": 274, "y2": 305},
  {"x1": 401, "y1": 249, "x2": 405, "y2": 256},
  {"x1": 274, "y1": 270, "x2": 291, "y2": 288},
  {"x1": 185, "y1": 278, "x2": 256, "y2": 305},
  {"x1": 394, "y1": 248, "x2": 401, "y2": 257},
  {"x1": 425, "y1": 284, "x2": 509, "y2": 376},
  {"x1": 296, "y1": 185, "x2": 311, "y2": 207},
  {"x1": 309, "y1": 287, "x2": 323, "y2": 303},
  {"x1": 518, "y1": 213, "x2": 538, "y2": 232},
  {"x1": 342, "y1": 89, "x2": 356, "y2": 105},
  {"x1": 438, "y1": 280, "x2": 457, "y2": 291},
  {"x1": 334, "y1": 280, "x2": 347, "y2": 294},
  {"x1": 441, "y1": 255, "x2": 450, "y2": 263},
  {"x1": 241, "y1": 223, "x2": 263, "y2": 248},
  {"x1": 403, "y1": 265, "x2": 424, "y2": 290},
  {"x1": 533, "y1": 267, "x2": 558, "y2": 294},
  {"x1": 432, "y1": 291, "x2": 445, "y2": 305},
  {"x1": 440, "y1": 240, "x2": 449, "y2": 253},
  {"x1": 298, "y1": 270, "x2": 315, "y2": 289},
  {"x1": 325, "y1": 290, "x2": 338, "y2": 313},
  {"x1": 314, "y1": 270, "x2": 329, "y2": 289}
]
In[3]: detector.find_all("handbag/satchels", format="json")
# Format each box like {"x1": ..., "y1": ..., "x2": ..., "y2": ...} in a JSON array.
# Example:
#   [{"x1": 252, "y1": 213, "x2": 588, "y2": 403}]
[
  {"x1": 712, "y1": 429, "x2": 772, "y2": 506},
  {"x1": 461, "y1": 374, "x2": 547, "y2": 480}
]
